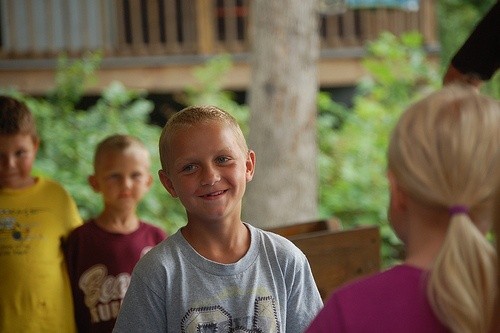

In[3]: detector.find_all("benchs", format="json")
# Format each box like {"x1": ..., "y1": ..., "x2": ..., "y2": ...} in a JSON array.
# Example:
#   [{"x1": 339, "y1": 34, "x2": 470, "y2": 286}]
[{"x1": 264, "y1": 219, "x2": 381, "y2": 304}]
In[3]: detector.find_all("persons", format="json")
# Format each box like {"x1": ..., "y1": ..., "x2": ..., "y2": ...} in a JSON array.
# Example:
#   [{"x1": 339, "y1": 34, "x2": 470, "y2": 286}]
[
  {"x1": 0, "y1": 94, "x2": 85, "y2": 333},
  {"x1": 301, "y1": 84, "x2": 500, "y2": 333},
  {"x1": 112, "y1": 105, "x2": 324, "y2": 333},
  {"x1": 65, "y1": 135, "x2": 168, "y2": 333},
  {"x1": 442, "y1": 0, "x2": 500, "y2": 92}
]
